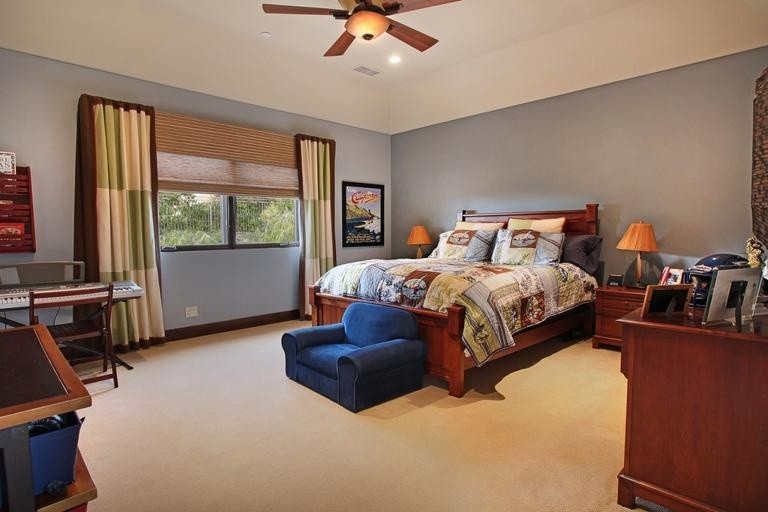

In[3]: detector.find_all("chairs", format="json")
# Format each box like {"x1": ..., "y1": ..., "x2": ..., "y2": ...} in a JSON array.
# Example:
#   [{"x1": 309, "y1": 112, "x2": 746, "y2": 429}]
[
  {"x1": 29, "y1": 283, "x2": 118, "y2": 388},
  {"x1": 281, "y1": 301, "x2": 426, "y2": 414}
]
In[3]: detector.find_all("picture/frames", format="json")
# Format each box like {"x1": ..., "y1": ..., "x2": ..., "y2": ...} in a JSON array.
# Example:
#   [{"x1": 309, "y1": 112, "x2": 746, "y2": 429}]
[
  {"x1": 640, "y1": 284, "x2": 694, "y2": 322},
  {"x1": 702, "y1": 266, "x2": 763, "y2": 333},
  {"x1": 341, "y1": 180, "x2": 385, "y2": 247}
]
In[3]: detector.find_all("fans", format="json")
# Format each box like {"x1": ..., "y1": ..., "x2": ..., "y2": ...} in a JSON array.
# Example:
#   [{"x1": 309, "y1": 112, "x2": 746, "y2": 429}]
[{"x1": 262, "y1": 0, "x2": 463, "y2": 56}]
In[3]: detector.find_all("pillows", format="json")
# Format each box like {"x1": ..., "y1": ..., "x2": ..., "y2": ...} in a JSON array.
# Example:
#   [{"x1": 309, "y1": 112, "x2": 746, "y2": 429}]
[{"x1": 428, "y1": 217, "x2": 603, "y2": 275}]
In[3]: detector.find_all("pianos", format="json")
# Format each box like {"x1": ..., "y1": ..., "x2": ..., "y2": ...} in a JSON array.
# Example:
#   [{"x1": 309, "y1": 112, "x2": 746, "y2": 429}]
[{"x1": 0, "y1": 281, "x2": 145, "y2": 311}]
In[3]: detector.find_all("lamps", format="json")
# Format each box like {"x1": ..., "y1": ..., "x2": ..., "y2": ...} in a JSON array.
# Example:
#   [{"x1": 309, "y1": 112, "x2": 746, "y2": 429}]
[
  {"x1": 406, "y1": 224, "x2": 432, "y2": 259},
  {"x1": 344, "y1": 10, "x2": 390, "y2": 40},
  {"x1": 615, "y1": 220, "x2": 659, "y2": 282}
]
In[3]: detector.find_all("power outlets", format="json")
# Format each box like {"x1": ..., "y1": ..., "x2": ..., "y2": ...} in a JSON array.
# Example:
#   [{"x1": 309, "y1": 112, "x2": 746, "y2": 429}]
[{"x1": 184, "y1": 306, "x2": 198, "y2": 318}]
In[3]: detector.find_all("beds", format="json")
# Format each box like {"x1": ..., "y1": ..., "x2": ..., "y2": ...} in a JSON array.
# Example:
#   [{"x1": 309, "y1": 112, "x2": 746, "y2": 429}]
[{"x1": 307, "y1": 203, "x2": 599, "y2": 398}]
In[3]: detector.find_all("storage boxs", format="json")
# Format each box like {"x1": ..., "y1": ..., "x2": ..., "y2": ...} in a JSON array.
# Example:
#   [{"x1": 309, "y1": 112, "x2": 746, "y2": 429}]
[{"x1": 28, "y1": 410, "x2": 85, "y2": 497}]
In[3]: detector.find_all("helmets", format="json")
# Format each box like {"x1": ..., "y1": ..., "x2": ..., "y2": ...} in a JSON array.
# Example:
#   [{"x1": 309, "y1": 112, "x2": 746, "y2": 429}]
[{"x1": 684, "y1": 253, "x2": 749, "y2": 308}]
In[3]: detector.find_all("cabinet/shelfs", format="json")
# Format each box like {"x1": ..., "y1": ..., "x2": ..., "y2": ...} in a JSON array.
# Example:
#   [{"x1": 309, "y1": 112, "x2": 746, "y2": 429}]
[
  {"x1": 592, "y1": 287, "x2": 646, "y2": 349},
  {"x1": 614, "y1": 306, "x2": 768, "y2": 512},
  {"x1": 0, "y1": 324, "x2": 97, "y2": 512},
  {"x1": 0, "y1": 166, "x2": 36, "y2": 252}
]
